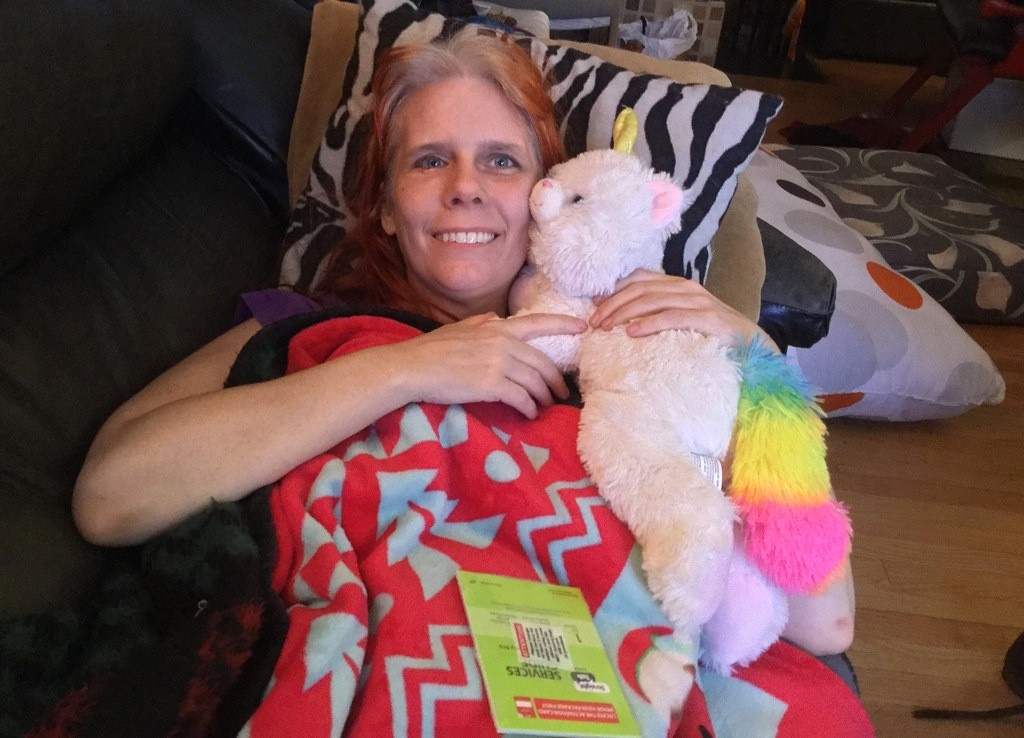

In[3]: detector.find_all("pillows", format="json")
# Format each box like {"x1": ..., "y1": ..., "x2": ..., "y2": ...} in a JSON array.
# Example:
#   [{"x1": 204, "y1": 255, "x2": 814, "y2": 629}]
[
  {"x1": 771, "y1": 145, "x2": 1024, "y2": 322},
  {"x1": 282, "y1": 0, "x2": 782, "y2": 299},
  {"x1": 286, "y1": 0, "x2": 766, "y2": 323},
  {"x1": 742, "y1": 150, "x2": 1007, "y2": 419}
]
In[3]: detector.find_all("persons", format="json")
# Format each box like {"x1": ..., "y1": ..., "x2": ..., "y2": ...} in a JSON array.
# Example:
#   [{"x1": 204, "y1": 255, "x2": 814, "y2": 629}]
[{"x1": 72, "y1": 37, "x2": 875, "y2": 738}]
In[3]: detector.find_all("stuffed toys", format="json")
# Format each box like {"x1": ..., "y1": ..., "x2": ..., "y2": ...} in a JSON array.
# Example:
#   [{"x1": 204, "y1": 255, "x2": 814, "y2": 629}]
[{"x1": 490, "y1": 104, "x2": 858, "y2": 674}]
